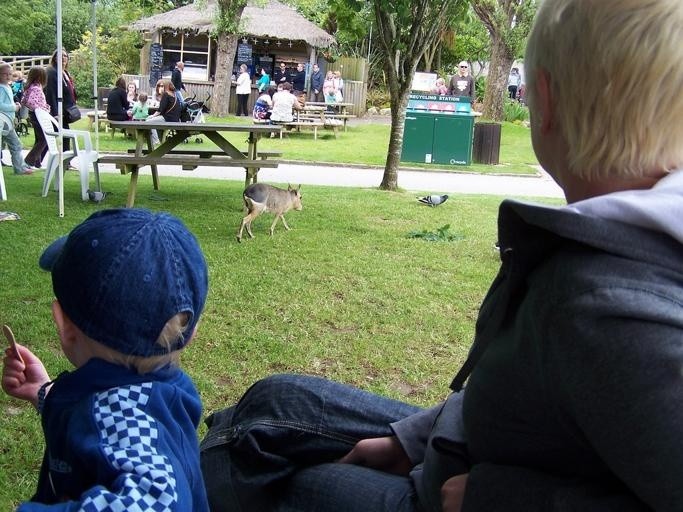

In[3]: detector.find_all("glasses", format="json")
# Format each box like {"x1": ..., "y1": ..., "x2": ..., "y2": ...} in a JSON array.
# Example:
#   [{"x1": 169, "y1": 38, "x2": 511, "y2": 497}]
[
  {"x1": 460, "y1": 66, "x2": 467, "y2": 68},
  {"x1": 157, "y1": 85, "x2": 164, "y2": 87}
]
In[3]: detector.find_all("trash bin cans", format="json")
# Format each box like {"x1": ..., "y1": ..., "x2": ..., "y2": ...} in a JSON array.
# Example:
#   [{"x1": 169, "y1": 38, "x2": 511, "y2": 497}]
[
  {"x1": 399, "y1": 94, "x2": 481, "y2": 167},
  {"x1": 471, "y1": 122, "x2": 501, "y2": 165}
]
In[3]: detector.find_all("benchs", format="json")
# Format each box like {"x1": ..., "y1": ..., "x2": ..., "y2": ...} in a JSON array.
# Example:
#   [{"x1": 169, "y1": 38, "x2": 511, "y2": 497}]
[
  {"x1": 95, "y1": 154, "x2": 280, "y2": 221},
  {"x1": 253, "y1": 99, "x2": 357, "y2": 141},
  {"x1": 127, "y1": 144, "x2": 281, "y2": 190},
  {"x1": 85, "y1": 104, "x2": 161, "y2": 139}
]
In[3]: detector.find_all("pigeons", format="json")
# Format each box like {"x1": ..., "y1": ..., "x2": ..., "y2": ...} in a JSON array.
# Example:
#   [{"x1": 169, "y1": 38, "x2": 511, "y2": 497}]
[
  {"x1": 415, "y1": 194, "x2": 449, "y2": 208},
  {"x1": 86, "y1": 188, "x2": 114, "y2": 206}
]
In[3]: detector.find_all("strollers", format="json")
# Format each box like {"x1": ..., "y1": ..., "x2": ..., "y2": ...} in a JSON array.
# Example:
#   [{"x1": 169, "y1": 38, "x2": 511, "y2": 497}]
[
  {"x1": 165, "y1": 89, "x2": 211, "y2": 145},
  {"x1": 10, "y1": 79, "x2": 28, "y2": 138}
]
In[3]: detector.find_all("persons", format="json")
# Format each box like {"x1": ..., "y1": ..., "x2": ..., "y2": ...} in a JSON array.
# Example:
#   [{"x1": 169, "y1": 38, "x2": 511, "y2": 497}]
[
  {"x1": 0, "y1": 205, "x2": 211, "y2": 512},
  {"x1": 203, "y1": 1, "x2": 683, "y2": 512},
  {"x1": 508, "y1": 67, "x2": 521, "y2": 98},
  {"x1": 44, "y1": 47, "x2": 78, "y2": 171},
  {"x1": 447, "y1": 60, "x2": 475, "y2": 105},
  {"x1": 0, "y1": 60, "x2": 34, "y2": 174},
  {"x1": 20, "y1": 64, "x2": 51, "y2": 168},
  {"x1": 106, "y1": 60, "x2": 186, "y2": 144},
  {"x1": 8, "y1": 70, "x2": 27, "y2": 116},
  {"x1": 232, "y1": 61, "x2": 346, "y2": 124}
]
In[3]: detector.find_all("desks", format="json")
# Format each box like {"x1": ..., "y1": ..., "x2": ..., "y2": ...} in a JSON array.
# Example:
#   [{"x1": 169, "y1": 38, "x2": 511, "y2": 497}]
[{"x1": 105, "y1": 118, "x2": 284, "y2": 214}]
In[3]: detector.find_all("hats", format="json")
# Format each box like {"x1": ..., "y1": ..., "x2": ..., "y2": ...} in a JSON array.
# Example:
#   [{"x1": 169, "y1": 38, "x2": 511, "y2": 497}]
[{"x1": 38, "y1": 207, "x2": 209, "y2": 358}]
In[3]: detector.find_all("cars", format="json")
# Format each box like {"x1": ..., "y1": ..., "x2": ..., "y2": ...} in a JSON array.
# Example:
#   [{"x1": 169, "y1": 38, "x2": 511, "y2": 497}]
[{"x1": 506, "y1": 62, "x2": 526, "y2": 106}]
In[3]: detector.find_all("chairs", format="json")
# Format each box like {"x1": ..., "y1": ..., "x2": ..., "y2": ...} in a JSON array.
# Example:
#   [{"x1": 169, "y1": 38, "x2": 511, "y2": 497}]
[{"x1": 32, "y1": 109, "x2": 102, "y2": 202}]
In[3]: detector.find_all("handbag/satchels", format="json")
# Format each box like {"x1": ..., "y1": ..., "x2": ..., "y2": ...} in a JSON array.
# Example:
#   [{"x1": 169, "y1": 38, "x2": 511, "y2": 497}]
[{"x1": 64, "y1": 105, "x2": 82, "y2": 124}]
